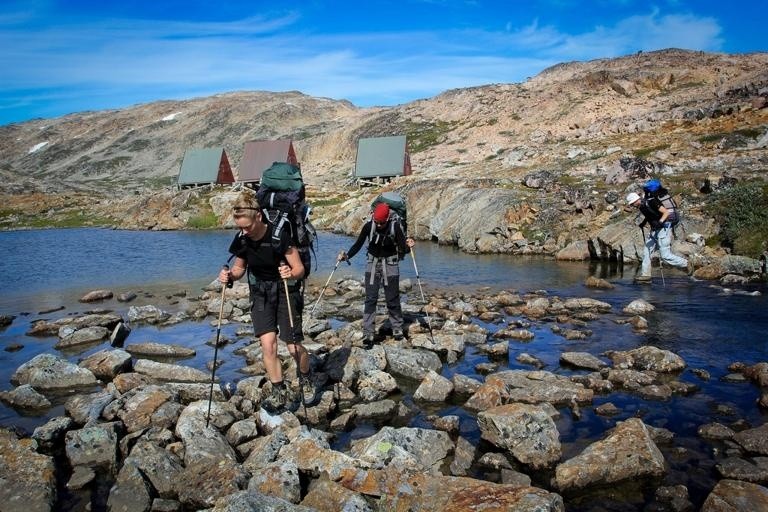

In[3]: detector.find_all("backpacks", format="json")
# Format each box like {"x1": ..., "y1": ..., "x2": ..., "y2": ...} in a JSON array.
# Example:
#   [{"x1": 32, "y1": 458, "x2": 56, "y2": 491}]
[
  {"x1": 237, "y1": 162, "x2": 311, "y2": 312},
  {"x1": 369, "y1": 191, "x2": 409, "y2": 286},
  {"x1": 637, "y1": 179, "x2": 679, "y2": 228}
]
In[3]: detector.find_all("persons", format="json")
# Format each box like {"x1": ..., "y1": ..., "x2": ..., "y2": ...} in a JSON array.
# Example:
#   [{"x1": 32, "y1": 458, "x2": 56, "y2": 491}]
[
  {"x1": 626, "y1": 192, "x2": 695, "y2": 285},
  {"x1": 337, "y1": 204, "x2": 415, "y2": 350},
  {"x1": 217, "y1": 192, "x2": 318, "y2": 413}
]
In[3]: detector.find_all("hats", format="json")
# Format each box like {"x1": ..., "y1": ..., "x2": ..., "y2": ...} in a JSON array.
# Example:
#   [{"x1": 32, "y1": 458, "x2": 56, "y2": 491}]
[
  {"x1": 626, "y1": 192, "x2": 641, "y2": 209},
  {"x1": 373, "y1": 203, "x2": 390, "y2": 222}
]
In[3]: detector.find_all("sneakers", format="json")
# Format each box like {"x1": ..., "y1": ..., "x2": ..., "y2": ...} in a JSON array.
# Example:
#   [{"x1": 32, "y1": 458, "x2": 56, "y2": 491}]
[
  {"x1": 360, "y1": 334, "x2": 375, "y2": 349},
  {"x1": 392, "y1": 326, "x2": 404, "y2": 339},
  {"x1": 298, "y1": 366, "x2": 316, "y2": 406},
  {"x1": 686, "y1": 260, "x2": 694, "y2": 277},
  {"x1": 261, "y1": 385, "x2": 287, "y2": 412},
  {"x1": 634, "y1": 275, "x2": 651, "y2": 282}
]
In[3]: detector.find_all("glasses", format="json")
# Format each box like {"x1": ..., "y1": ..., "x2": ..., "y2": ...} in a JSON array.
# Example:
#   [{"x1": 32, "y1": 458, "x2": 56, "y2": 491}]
[{"x1": 373, "y1": 221, "x2": 387, "y2": 225}]
